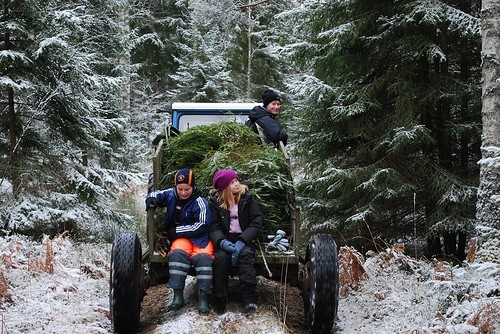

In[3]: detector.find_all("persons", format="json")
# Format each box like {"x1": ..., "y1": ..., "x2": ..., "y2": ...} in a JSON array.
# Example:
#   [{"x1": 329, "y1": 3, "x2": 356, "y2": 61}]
[
  {"x1": 146, "y1": 169, "x2": 215, "y2": 316},
  {"x1": 209, "y1": 169, "x2": 264, "y2": 318},
  {"x1": 246, "y1": 90, "x2": 288, "y2": 149}
]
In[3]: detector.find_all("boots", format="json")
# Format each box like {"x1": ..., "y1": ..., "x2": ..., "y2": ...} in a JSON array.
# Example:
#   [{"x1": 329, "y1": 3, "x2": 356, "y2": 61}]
[
  {"x1": 168, "y1": 289, "x2": 185, "y2": 309},
  {"x1": 197, "y1": 289, "x2": 211, "y2": 316}
]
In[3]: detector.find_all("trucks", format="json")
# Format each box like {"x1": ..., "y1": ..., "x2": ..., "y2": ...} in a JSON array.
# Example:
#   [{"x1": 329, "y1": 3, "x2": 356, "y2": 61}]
[{"x1": 108, "y1": 102, "x2": 340, "y2": 334}]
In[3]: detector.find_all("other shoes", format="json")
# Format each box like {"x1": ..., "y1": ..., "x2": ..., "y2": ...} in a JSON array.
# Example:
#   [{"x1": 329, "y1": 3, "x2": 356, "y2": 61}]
[
  {"x1": 241, "y1": 296, "x2": 258, "y2": 309},
  {"x1": 216, "y1": 296, "x2": 226, "y2": 313}
]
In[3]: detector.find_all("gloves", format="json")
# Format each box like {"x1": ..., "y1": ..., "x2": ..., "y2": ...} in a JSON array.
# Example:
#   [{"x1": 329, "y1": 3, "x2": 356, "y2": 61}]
[
  {"x1": 145, "y1": 198, "x2": 158, "y2": 211},
  {"x1": 268, "y1": 229, "x2": 289, "y2": 251},
  {"x1": 165, "y1": 227, "x2": 177, "y2": 241},
  {"x1": 220, "y1": 238, "x2": 245, "y2": 265}
]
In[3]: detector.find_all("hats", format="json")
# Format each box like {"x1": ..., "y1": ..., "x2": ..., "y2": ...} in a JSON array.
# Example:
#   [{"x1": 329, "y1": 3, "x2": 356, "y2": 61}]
[
  {"x1": 213, "y1": 169, "x2": 238, "y2": 192},
  {"x1": 174, "y1": 169, "x2": 195, "y2": 187},
  {"x1": 262, "y1": 90, "x2": 281, "y2": 107}
]
